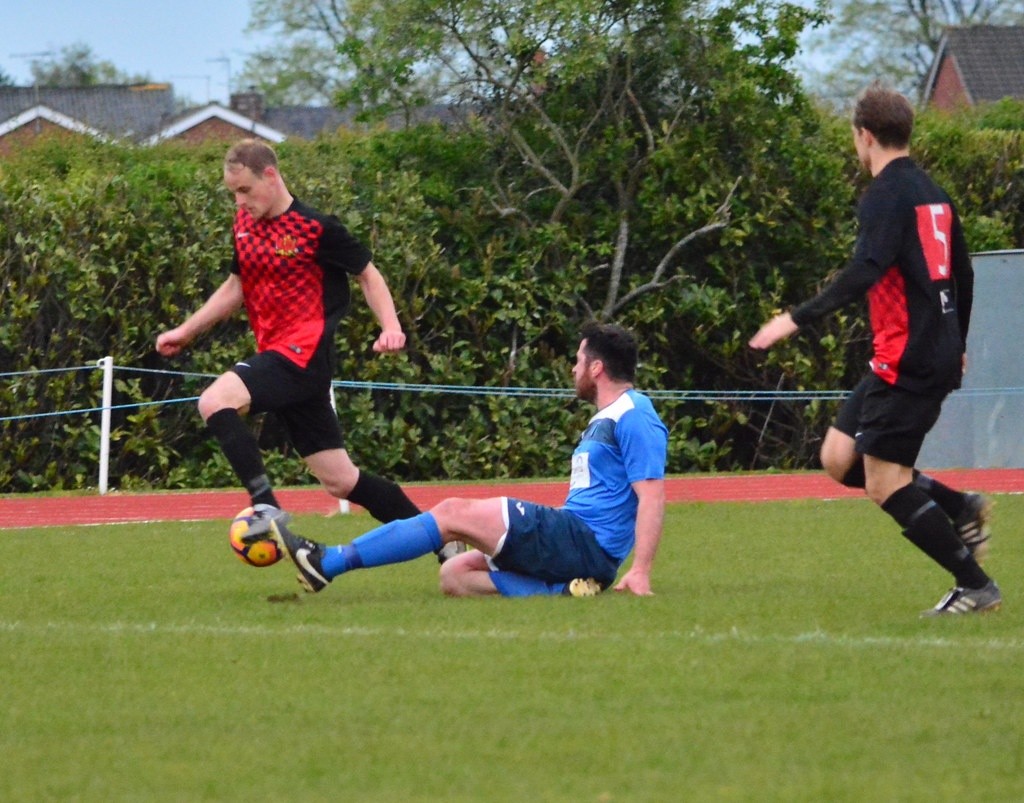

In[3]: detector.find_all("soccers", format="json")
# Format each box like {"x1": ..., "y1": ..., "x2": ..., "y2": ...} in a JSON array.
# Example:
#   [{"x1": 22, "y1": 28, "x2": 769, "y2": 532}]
[{"x1": 228, "y1": 502, "x2": 291, "y2": 568}]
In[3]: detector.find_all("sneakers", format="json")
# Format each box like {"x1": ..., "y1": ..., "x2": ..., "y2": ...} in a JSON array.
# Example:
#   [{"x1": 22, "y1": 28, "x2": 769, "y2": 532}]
[
  {"x1": 270, "y1": 518, "x2": 333, "y2": 593},
  {"x1": 562, "y1": 577, "x2": 601, "y2": 597},
  {"x1": 239, "y1": 507, "x2": 289, "y2": 545},
  {"x1": 919, "y1": 579, "x2": 1002, "y2": 619},
  {"x1": 438, "y1": 540, "x2": 468, "y2": 566},
  {"x1": 953, "y1": 491, "x2": 997, "y2": 568}
]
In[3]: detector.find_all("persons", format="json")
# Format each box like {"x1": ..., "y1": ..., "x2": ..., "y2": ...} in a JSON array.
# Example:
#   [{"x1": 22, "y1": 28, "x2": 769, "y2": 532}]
[
  {"x1": 153, "y1": 139, "x2": 466, "y2": 564},
  {"x1": 268, "y1": 322, "x2": 668, "y2": 597},
  {"x1": 747, "y1": 90, "x2": 1004, "y2": 617}
]
